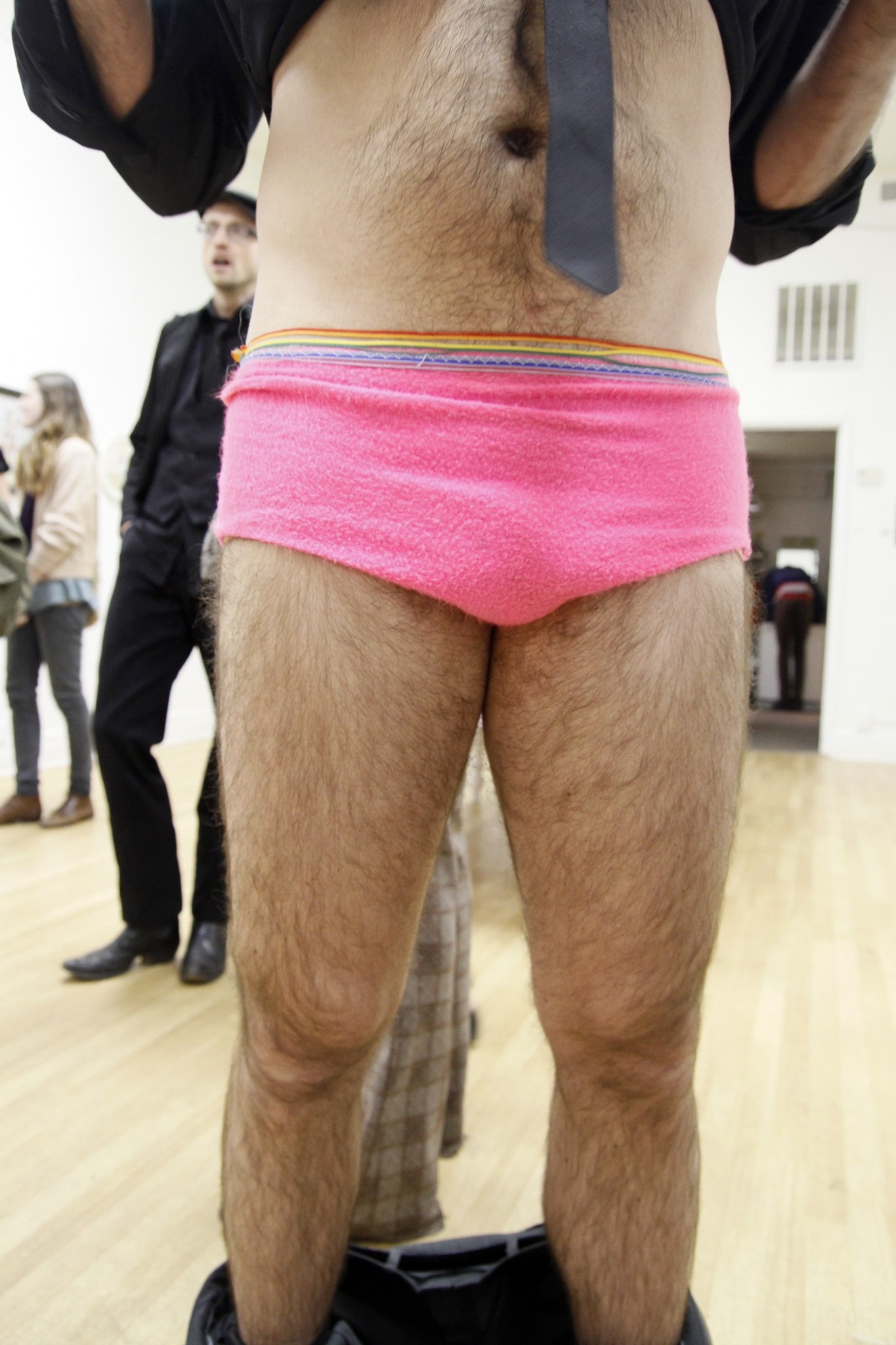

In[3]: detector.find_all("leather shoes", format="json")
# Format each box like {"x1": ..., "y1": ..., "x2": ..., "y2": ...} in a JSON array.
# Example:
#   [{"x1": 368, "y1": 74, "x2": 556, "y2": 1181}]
[
  {"x1": 0, "y1": 796, "x2": 41, "y2": 825},
  {"x1": 42, "y1": 796, "x2": 94, "y2": 828},
  {"x1": 62, "y1": 922, "x2": 179, "y2": 978},
  {"x1": 183, "y1": 918, "x2": 230, "y2": 981}
]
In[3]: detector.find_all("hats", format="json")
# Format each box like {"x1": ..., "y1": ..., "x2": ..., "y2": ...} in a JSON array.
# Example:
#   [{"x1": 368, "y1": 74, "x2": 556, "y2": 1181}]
[{"x1": 197, "y1": 188, "x2": 257, "y2": 220}]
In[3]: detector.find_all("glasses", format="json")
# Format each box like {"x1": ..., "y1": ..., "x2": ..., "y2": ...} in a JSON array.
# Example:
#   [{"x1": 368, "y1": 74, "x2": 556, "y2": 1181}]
[{"x1": 197, "y1": 221, "x2": 255, "y2": 242}]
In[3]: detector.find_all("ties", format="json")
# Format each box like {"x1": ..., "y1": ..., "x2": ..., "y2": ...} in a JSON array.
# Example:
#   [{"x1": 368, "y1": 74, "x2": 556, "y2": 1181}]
[{"x1": 545, "y1": 0, "x2": 619, "y2": 295}]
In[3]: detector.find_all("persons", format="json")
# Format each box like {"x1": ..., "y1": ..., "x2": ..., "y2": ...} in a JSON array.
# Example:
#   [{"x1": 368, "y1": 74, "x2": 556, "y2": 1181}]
[
  {"x1": 349, "y1": 764, "x2": 473, "y2": 1243},
  {"x1": 757, "y1": 566, "x2": 816, "y2": 711},
  {"x1": 61, "y1": 193, "x2": 258, "y2": 985},
  {"x1": 13, "y1": 0, "x2": 896, "y2": 1345},
  {"x1": 0, "y1": 374, "x2": 97, "y2": 827}
]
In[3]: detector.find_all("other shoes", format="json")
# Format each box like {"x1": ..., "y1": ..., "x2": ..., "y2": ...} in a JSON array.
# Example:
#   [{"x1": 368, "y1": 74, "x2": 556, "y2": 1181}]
[{"x1": 776, "y1": 699, "x2": 802, "y2": 711}]
